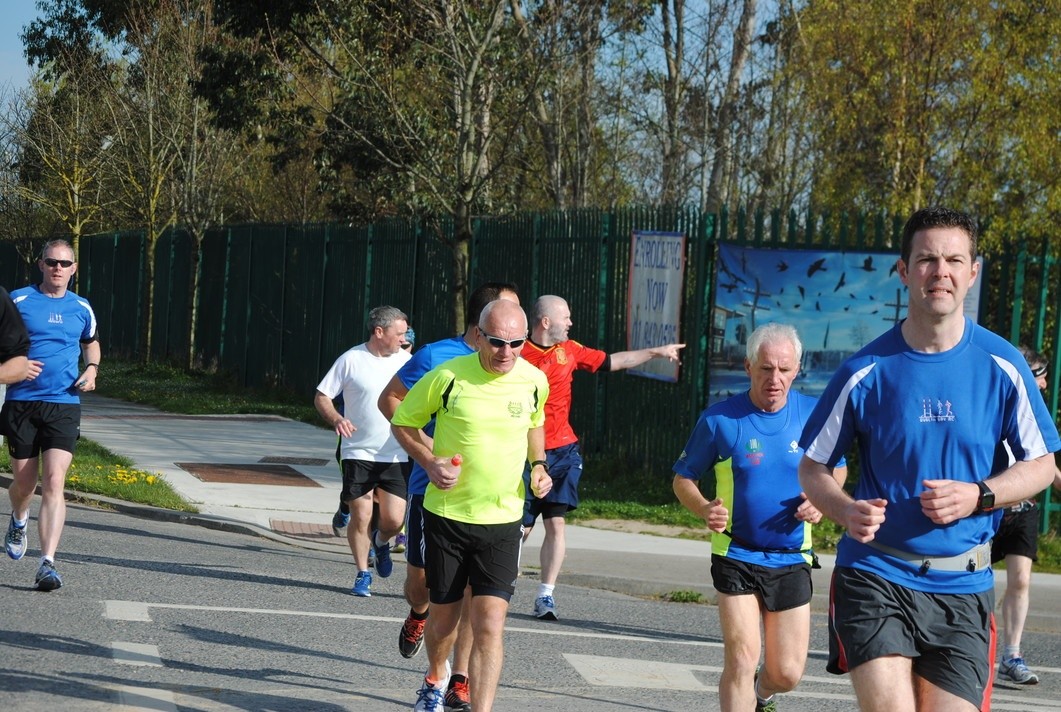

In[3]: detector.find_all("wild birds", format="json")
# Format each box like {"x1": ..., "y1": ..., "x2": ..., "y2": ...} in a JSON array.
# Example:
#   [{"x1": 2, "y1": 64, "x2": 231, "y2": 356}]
[
  {"x1": 798, "y1": 285, "x2": 805, "y2": 301},
  {"x1": 850, "y1": 294, "x2": 856, "y2": 299},
  {"x1": 869, "y1": 294, "x2": 875, "y2": 300},
  {"x1": 834, "y1": 272, "x2": 846, "y2": 292},
  {"x1": 817, "y1": 292, "x2": 821, "y2": 295},
  {"x1": 793, "y1": 304, "x2": 800, "y2": 308},
  {"x1": 779, "y1": 286, "x2": 784, "y2": 294},
  {"x1": 871, "y1": 310, "x2": 878, "y2": 314},
  {"x1": 844, "y1": 305, "x2": 850, "y2": 311},
  {"x1": 777, "y1": 300, "x2": 780, "y2": 306},
  {"x1": 807, "y1": 258, "x2": 827, "y2": 278},
  {"x1": 720, "y1": 283, "x2": 739, "y2": 293},
  {"x1": 815, "y1": 300, "x2": 820, "y2": 312},
  {"x1": 741, "y1": 252, "x2": 748, "y2": 274},
  {"x1": 731, "y1": 272, "x2": 747, "y2": 286},
  {"x1": 851, "y1": 255, "x2": 877, "y2": 271},
  {"x1": 889, "y1": 263, "x2": 898, "y2": 278},
  {"x1": 719, "y1": 256, "x2": 731, "y2": 279},
  {"x1": 776, "y1": 260, "x2": 788, "y2": 272}
]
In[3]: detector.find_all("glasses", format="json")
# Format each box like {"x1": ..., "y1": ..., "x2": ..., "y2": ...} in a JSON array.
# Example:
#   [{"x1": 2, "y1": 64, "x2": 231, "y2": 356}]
[
  {"x1": 477, "y1": 326, "x2": 528, "y2": 349},
  {"x1": 43, "y1": 258, "x2": 75, "y2": 268},
  {"x1": 401, "y1": 344, "x2": 411, "y2": 349}
]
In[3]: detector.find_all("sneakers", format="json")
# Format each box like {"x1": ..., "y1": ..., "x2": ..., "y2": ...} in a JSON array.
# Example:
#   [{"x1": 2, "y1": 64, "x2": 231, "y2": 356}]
[
  {"x1": 332, "y1": 503, "x2": 351, "y2": 537},
  {"x1": 392, "y1": 530, "x2": 406, "y2": 553},
  {"x1": 368, "y1": 545, "x2": 376, "y2": 566},
  {"x1": 34, "y1": 565, "x2": 63, "y2": 591},
  {"x1": 753, "y1": 664, "x2": 776, "y2": 712},
  {"x1": 349, "y1": 572, "x2": 372, "y2": 597},
  {"x1": 443, "y1": 674, "x2": 471, "y2": 712},
  {"x1": 399, "y1": 604, "x2": 429, "y2": 659},
  {"x1": 5, "y1": 508, "x2": 29, "y2": 560},
  {"x1": 997, "y1": 654, "x2": 1040, "y2": 685},
  {"x1": 371, "y1": 529, "x2": 393, "y2": 578},
  {"x1": 414, "y1": 659, "x2": 451, "y2": 712},
  {"x1": 533, "y1": 595, "x2": 559, "y2": 621}
]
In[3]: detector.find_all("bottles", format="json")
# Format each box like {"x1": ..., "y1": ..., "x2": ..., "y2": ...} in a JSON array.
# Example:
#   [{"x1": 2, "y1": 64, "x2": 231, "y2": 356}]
[{"x1": 445, "y1": 453, "x2": 463, "y2": 478}]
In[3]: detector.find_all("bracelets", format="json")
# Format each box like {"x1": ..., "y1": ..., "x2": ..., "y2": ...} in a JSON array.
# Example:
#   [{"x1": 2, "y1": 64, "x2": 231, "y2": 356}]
[{"x1": 85, "y1": 363, "x2": 101, "y2": 372}]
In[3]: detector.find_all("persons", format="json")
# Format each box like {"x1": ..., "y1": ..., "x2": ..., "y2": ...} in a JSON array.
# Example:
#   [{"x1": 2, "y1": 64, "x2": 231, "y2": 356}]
[
  {"x1": 353, "y1": 322, "x2": 418, "y2": 566},
  {"x1": 376, "y1": 279, "x2": 519, "y2": 711},
  {"x1": 312, "y1": 303, "x2": 415, "y2": 600},
  {"x1": 782, "y1": 200, "x2": 1056, "y2": 712},
  {"x1": 326, "y1": 428, "x2": 351, "y2": 536},
  {"x1": 0, "y1": 239, "x2": 103, "y2": 592},
  {"x1": 672, "y1": 326, "x2": 848, "y2": 712},
  {"x1": 990, "y1": 341, "x2": 1061, "y2": 687},
  {"x1": 387, "y1": 304, "x2": 554, "y2": 712},
  {"x1": 520, "y1": 295, "x2": 687, "y2": 621},
  {"x1": 0, "y1": 285, "x2": 31, "y2": 385}
]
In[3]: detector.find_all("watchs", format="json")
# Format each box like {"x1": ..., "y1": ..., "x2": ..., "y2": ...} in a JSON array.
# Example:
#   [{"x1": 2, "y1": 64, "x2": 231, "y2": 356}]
[
  {"x1": 969, "y1": 474, "x2": 995, "y2": 514},
  {"x1": 530, "y1": 458, "x2": 549, "y2": 475}
]
